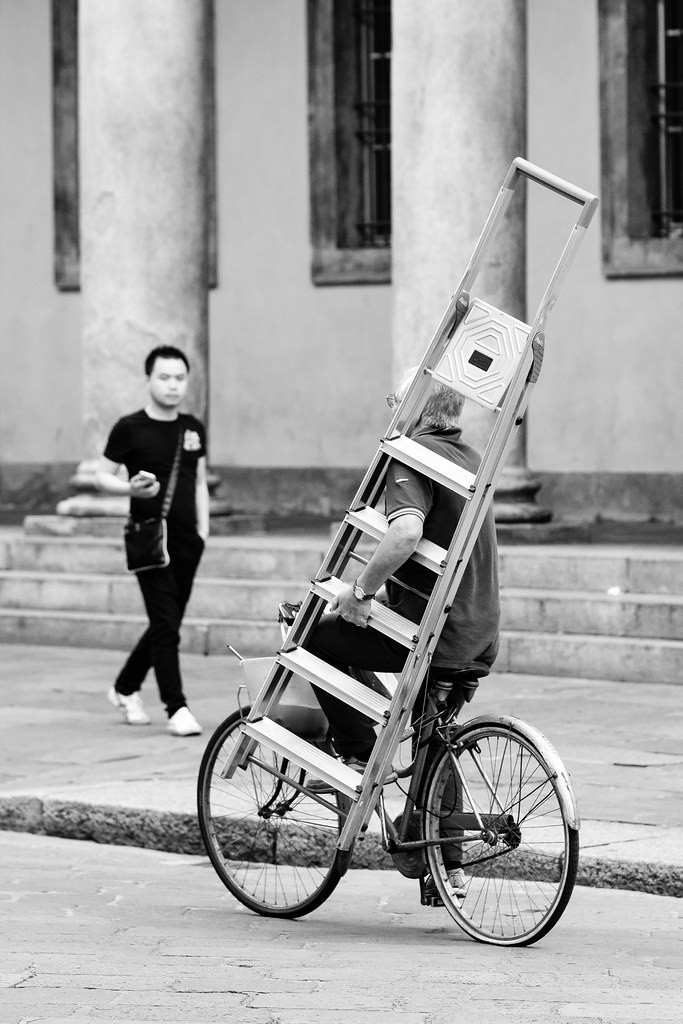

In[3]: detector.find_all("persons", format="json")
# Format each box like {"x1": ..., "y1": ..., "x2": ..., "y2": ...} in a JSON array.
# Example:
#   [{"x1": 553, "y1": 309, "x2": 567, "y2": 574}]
[
  {"x1": 299, "y1": 365, "x2": 502, "y2": 910},
  {"x1": 93, "y1": 346, "x2": 210, "y2": 737}
]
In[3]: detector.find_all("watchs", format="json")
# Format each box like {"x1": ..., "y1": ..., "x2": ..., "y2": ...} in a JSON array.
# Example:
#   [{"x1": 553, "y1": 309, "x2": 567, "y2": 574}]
[{"x1": 353, "y1": 579, "x2": 377, "y2": 602}]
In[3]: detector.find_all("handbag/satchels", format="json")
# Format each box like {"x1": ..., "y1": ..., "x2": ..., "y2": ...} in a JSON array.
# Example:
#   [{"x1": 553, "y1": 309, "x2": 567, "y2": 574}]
[{"x1": 123, "y1": 517, "x2": 170, "y2": 575}]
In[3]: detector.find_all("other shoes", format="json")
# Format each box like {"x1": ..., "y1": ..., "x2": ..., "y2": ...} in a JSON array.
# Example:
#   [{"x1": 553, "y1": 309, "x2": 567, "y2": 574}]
[
  {"x1": 425, "y1": 868, "x2": 467, "y2": 899},
  {"x1": 306, "y1": 755, "x2": 399, "y2": 793}
]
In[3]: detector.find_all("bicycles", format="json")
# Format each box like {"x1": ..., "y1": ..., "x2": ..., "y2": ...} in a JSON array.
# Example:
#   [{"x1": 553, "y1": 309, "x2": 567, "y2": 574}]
[{"x1": 195, "y1": 599, "x2": 583, "y2": 950}]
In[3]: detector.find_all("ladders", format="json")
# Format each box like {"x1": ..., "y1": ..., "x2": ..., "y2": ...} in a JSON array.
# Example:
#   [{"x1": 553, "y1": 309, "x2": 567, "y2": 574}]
[{"x1": 219, "y1": 156, "x2": 602, "y2": 875}]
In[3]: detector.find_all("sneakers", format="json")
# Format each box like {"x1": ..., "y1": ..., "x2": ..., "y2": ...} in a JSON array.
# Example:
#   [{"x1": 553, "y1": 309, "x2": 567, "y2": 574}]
[
  {"x1": 105, "y1": 685, "x2": 151, "y2": 725},
  {"x1": 166, "y1": 707, "x2": 203, "y2": 737}
]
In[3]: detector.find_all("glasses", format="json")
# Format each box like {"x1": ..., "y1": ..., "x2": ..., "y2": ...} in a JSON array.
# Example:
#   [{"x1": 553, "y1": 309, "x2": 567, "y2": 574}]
[{"x1": 386, "y1": 392, "x2": 402, "y2": 409}]
